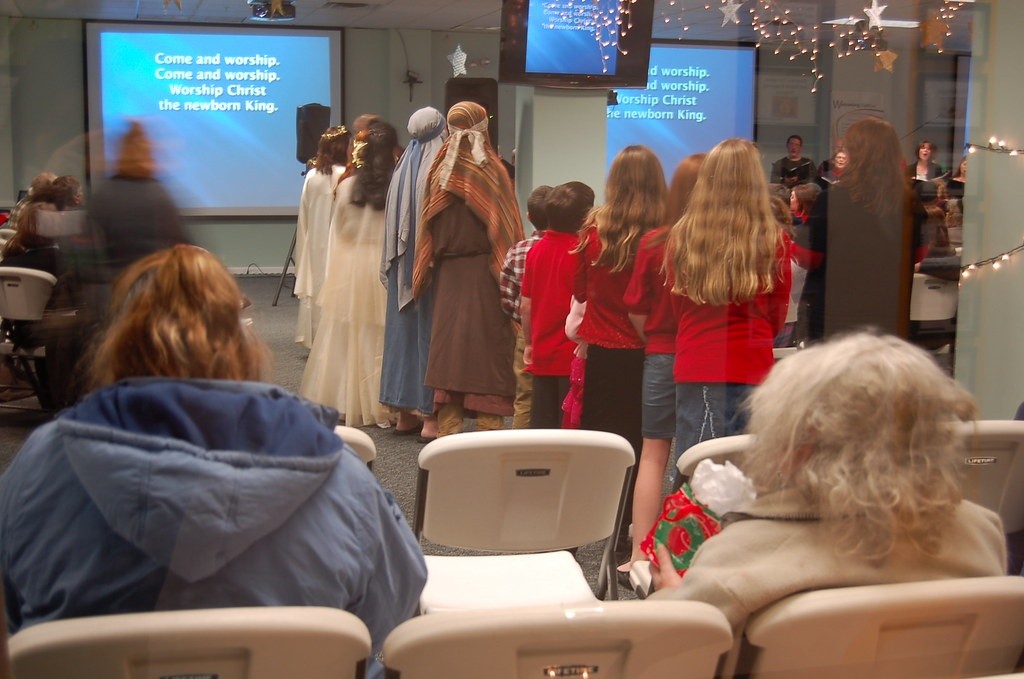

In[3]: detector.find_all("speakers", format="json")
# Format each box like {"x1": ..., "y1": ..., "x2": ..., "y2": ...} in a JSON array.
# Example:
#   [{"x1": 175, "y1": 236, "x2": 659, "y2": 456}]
[
  {"x1": 296, "y1": 103, "x2": 331, "y2": 164},
  {"x1": 445, "y1": 77, "x2": 498, "y2": 157}
]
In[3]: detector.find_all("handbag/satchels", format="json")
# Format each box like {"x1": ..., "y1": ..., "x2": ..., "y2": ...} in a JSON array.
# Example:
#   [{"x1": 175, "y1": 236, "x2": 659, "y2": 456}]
[{"x1": 638, "y1": 484, "x2": 723, "y2": 579}]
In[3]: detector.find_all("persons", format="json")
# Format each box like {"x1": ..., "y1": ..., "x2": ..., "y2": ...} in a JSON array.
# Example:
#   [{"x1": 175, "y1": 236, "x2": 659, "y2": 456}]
[
  {"x1": 10, "y1": 171, "x2": 81, "y2": 241},
  {"x1": 914, "y1": 255, "x2": 961, "y2": 283},
  {"x1": 566, "y1": 144, "x2": 669, "y2": 561},
  {"x1": 499, "y1": 186, "x2": 554, "y2": 429},
  {"x1": 415, "y1": 102, "x2": 527, "y2": 440},
  {"x1": 560, "y1": 294, "x2": 591, "y2": 428},
  {"x1": 293, "y1": 114, "x2": 407, "y2": 428},
  {"x1": 771, "y1": 116, "x2": 967, "y2": 251},
  {"x1": 0, "y1": 244, "x2": 427, "y2": 679},
  {"x1": 519, "y1": 181, "x2": 595, "y2": 430},
  {"x1": 4, "y1": 199, "x2": 76, "y2": 352},
  {"x1": 639, "y1": 333, "x2": 1009, "y2": 678},
  {"x1": 616, "y1": 153, "x2": 709, "y2": 586},
  {"x1": 767, "y1": 196, "x2": 809, "y2": 348},
  {"x1": 662, "y1": 138, "x2": 793, "y2": 492},
  {"x1": 379, "y1": 105, "x2": 448, "y2": 444},
  {"x1": 904, "y1": 205, "x2": 956, "y2": 350},
  {"x1": 82, "y1": 131, "x2": 191, "y2": 327}
]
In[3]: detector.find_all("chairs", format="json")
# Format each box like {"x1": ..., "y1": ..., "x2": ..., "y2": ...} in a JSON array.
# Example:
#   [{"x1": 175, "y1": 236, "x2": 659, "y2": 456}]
[
  {"x1": 950, "y1": 412, "x2": 1024, "y2": 538},
  {"x1": 7, "y1": 605, "x2": 371, "y2": 679},
  {"x1": 670, "y1": 432, "x2": 755, "y2": 500},
  {"x1": 0, "y1": 265, "x2": 76, "y2": 414},
  {"x1": 380, "y1": 600, "x2": 734, "y2": 679},
  {"x1": 333, "y1": 424, "x2": 376, "y2": 473},
  {"x1": 735, "y1": 575, "x2": 1024, "y2": 679},
  {"x1": 399, "y1": 430, "x2": 638, "y2": 614}
]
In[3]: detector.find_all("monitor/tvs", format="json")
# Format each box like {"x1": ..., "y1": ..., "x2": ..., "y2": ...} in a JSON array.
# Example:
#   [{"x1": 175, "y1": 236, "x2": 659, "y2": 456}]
[{"x1": 497, "y1": 0, "x2": 654, "y2": 90}]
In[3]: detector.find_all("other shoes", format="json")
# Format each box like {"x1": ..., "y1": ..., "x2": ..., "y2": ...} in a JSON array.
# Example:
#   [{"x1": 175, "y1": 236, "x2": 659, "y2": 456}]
[{"x1": 616, "y1": 561, "x2": 632, "y2": 589}]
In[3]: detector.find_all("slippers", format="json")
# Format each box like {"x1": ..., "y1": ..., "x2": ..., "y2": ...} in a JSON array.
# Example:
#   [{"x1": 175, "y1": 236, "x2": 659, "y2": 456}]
[
  {"x1": 416, "y1": 433, "x2": 437, "y2": 443},
  {"x1": 394, "y1": 428, "x2": 419, "y2": 436}
]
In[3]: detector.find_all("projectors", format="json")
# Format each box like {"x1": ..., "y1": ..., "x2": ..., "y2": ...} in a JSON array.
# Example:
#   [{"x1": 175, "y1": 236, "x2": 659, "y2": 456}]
[{"x1": 251, "y1": 3, "x2": 296, "y2": 21}]
